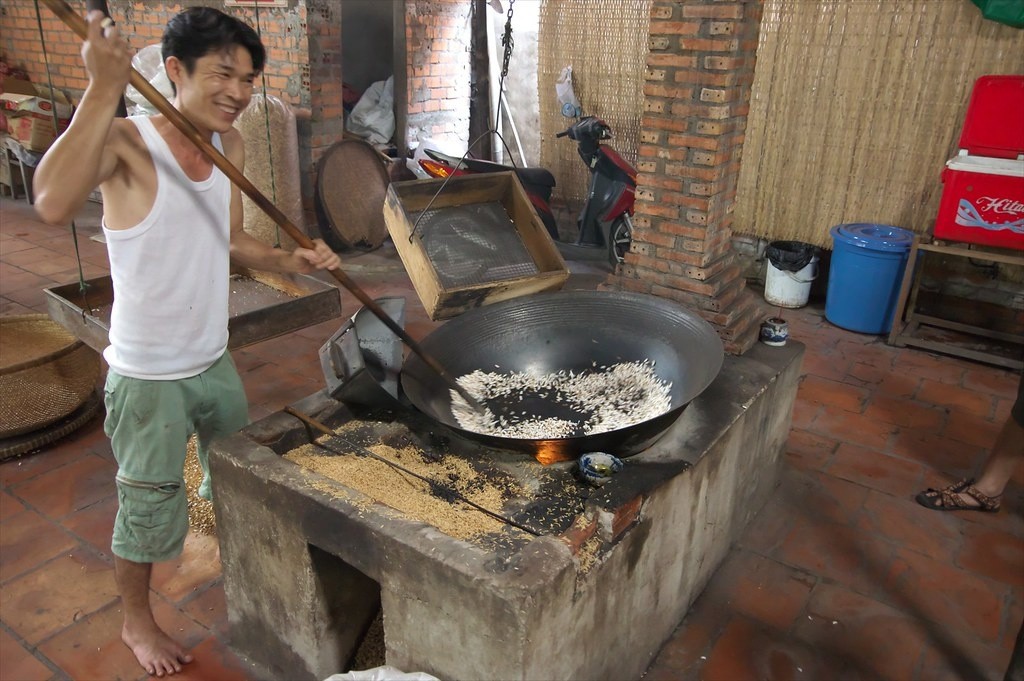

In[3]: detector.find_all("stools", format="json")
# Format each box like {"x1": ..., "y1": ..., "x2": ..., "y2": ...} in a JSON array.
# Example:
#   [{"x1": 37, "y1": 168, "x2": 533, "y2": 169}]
[{"x1": 4, "y1": 141, "x2": 54, "y2": 204}]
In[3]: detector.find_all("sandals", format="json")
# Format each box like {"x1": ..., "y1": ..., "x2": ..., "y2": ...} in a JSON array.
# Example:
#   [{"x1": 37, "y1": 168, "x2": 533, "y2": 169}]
[{"x1": 916, "y1": 476, "x2": 1001, "y2": 512}]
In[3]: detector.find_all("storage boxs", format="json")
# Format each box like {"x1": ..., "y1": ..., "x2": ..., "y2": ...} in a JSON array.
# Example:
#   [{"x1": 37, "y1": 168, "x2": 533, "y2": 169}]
[
  {"x1": 932, "y1": 75, "x2": 1024, "y2": 250},
  {"x1": 0, "y1": 146, "x2": 35, "y2": 186},
  {"x1": 0, "y1": 75, "x2": 73, "y2": 152}
]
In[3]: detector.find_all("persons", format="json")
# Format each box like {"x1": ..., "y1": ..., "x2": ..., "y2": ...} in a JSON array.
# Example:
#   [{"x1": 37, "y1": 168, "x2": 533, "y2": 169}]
[
  {"x1": 31, "y1": 5, "x2": 339, "y2": 677},
  {"x1": 916, "y1": 375, "x2": 1024, "y2": 512}
]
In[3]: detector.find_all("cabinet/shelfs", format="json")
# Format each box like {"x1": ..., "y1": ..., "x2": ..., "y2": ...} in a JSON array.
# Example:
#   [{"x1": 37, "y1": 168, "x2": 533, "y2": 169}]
[{"x1": 887, "y1": 233, "x2": 1024, "y2": 371}]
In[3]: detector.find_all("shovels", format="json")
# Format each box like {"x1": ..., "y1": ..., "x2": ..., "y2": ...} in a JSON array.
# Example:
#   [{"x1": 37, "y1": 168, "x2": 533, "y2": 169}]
[{"x1": 48, "y1": 0, "x2": 567, "y2": 426}]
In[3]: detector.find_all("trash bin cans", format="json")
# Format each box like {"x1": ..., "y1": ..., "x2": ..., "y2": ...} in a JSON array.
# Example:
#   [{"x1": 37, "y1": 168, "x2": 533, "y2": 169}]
[{"x1": 763, "y1": 239, "x2": 824, "y2": 310}]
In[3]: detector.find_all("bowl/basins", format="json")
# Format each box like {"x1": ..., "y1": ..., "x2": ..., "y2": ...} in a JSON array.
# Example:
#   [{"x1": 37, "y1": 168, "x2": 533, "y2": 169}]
[{"x1": 578, "y1": 451, "x2": 623, "y2": 487}]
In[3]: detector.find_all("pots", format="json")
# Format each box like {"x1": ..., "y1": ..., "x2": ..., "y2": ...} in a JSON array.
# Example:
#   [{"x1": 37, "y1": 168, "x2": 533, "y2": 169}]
[{"x1": 400, "y1": 289, "x2": 725, "y2": 466}]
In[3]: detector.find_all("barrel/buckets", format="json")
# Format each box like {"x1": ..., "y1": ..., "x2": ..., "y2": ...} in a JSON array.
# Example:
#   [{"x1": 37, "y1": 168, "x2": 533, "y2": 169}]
[{"x1": 824, "y1": 222, "x2": 915, "y2": 334}]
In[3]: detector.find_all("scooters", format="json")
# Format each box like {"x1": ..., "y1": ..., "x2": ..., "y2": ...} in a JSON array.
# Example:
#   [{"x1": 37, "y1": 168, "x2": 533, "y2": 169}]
[{"x1": 417, "y1": 102, "x2": 637, "y2": 271}]
